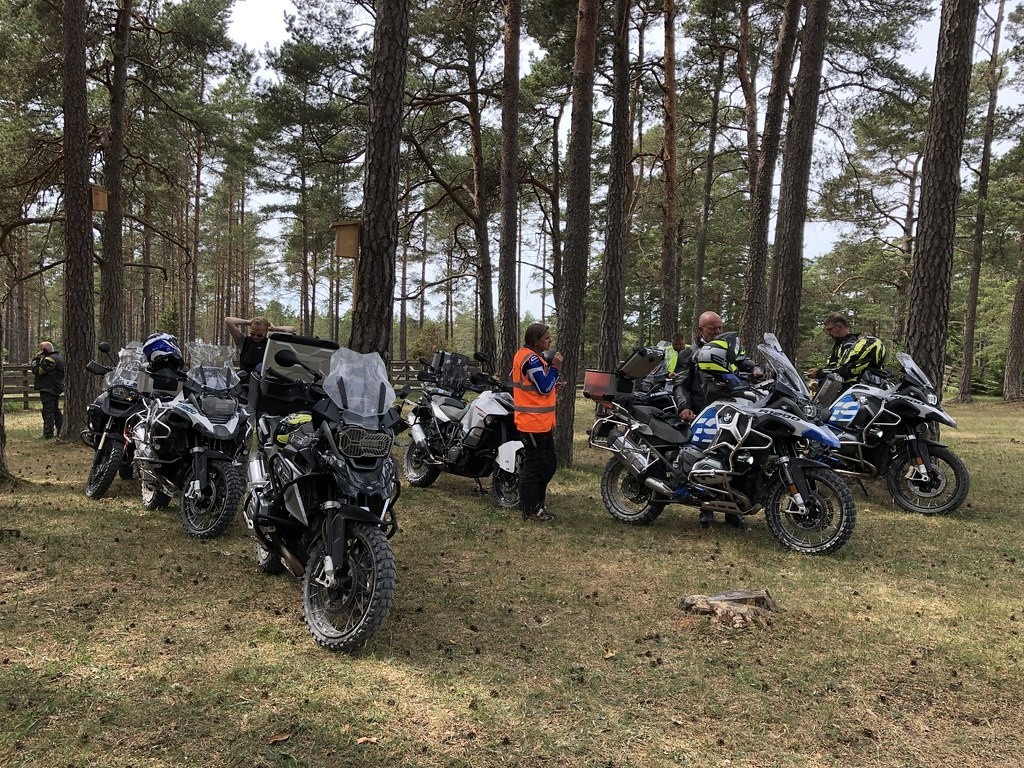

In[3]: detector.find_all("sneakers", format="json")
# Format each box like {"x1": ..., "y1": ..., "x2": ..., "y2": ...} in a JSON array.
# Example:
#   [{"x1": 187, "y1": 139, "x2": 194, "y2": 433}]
[{"x1": 521, "y1": 506, "x2": 554, "y2": 525}]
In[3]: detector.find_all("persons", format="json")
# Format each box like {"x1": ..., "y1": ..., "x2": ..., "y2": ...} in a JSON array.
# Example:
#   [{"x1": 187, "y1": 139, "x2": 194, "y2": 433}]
[
  {"x1": 673, "y1": 311, "x2": 762, "y2": 527},
  {"x1": 32, "y1": 342, "x2": 65, "y2": 440},
  {"x1": 804, "y1": 313, "x2": 886, "y2": 386},
  {"x1": 512, "y1": 323, "x2": 566, "y2": 523},
  {"x1": 225, "y1": 317, "x2": 296, "y2": 412},
  {"x1": 665, "y1": 331, "x2": 692, "y2": 379}
]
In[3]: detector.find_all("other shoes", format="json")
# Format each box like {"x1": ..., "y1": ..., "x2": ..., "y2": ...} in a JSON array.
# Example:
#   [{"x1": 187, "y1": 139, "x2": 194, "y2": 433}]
[
  {"x1": 35, "y1": 436, "x2": 47, "y2": 442},
  {"x1": 725, "y1": 514, "x2": 752, "y2": 527},
  {"x1": 699, "y1": 511, "x2": 714, "y2": 524}
]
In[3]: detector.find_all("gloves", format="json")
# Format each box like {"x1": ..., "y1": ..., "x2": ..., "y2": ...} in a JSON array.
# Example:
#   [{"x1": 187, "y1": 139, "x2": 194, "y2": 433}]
[{"x1": 649, "y1": 411, "x2": 683, "y2": 431}]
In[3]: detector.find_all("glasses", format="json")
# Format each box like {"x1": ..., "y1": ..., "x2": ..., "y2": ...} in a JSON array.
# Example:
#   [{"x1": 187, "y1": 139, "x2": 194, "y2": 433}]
[
  {"x1": 827, "y1": 324, "x2": 841, "y2": 333},
  {"x1": 250, "y1": 332, "x2": 266, "y2": 337}
]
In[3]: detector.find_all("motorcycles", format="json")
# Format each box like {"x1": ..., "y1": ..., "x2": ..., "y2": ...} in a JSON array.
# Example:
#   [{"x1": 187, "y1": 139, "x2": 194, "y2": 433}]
[
  {"x1": 584, "y1": 330, "x2": 858, "y2": 555},
  {"x1": 401, "y1": 348, "x2": 526, "y2": 508},
  {"x1": 797, "y1": 349, "x2": 970, "y2": 515},
  {"x1": 78, "y1": 333, "x2": 256, "y2": 540},
  {"x1": 238, "y1": 331, "x2": 435, "y2": 651}
]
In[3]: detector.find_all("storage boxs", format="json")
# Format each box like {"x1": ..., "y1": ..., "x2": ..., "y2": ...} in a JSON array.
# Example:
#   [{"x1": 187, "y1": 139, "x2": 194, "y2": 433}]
[
  {"x1": 583, "y1": 346, "x2": 666, "y2": 397},
  {"x1": 428, "y1": 349, "x2": 451, "y2": 375},
  {"x1": 248, "y1": 332, "x2": 340, "y2": 416}
]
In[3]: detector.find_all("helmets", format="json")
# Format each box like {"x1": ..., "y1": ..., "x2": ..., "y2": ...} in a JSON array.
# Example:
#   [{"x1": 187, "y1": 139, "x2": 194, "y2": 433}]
[
  {"x1": 143, "y1": 333, "x2": 184, "y2": 371},
  {"x1": 698, "y1": 339, "x2": 745, "y2": 374},
  {"x1": 273, "y1": 411, "x2": 312, "y2": 453},
  {"x1": 850, "y1": 335, "x2": 886, "y2": 369}
]
